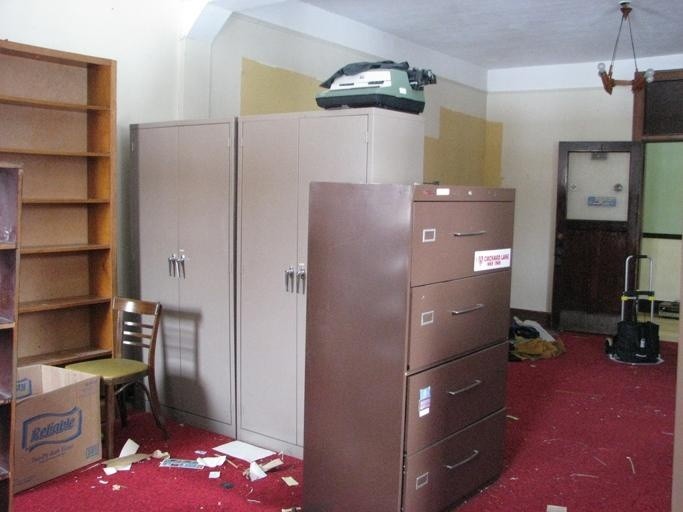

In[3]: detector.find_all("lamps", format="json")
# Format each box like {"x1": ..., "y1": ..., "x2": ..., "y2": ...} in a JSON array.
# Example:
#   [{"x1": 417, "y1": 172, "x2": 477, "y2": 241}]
[{"x1": 594, "y1": 2, "x2": 656, "y2": 96}]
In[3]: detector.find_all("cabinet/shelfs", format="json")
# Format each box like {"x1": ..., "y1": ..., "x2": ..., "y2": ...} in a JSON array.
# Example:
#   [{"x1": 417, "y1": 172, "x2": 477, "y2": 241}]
[
  {"x1": 236, "y1": 107, "x2": 423, "y2": 464},
  {"x1": 0, "y1": 161, "x2": 23, "y2": 512},
  {"x1": 127, "y1": 116, "x2": 237, "y2": 439},
  {"x1": 0, "y1": 36, "x2": 118, "y2": 425},
  {"x1": 300, "y1": 180, "x2": 517, "y2": 512}
]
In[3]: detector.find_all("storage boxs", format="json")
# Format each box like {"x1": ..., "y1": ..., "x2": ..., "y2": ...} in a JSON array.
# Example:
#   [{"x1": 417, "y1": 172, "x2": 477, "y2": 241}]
[{"x1": 10, "y1": 362, "x2": 102, "y2": 497}]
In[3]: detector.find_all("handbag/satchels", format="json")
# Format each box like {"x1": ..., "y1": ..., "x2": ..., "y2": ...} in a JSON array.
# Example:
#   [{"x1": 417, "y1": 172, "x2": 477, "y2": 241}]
[{"x1": 615, "y1": 320, "x2": 660, "y2": 364}]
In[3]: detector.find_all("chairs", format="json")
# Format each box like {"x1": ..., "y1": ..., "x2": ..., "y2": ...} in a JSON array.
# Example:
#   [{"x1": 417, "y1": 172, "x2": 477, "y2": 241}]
[{"x1": 60, "y1": 296, "x2": 166, "y2": 461}]
